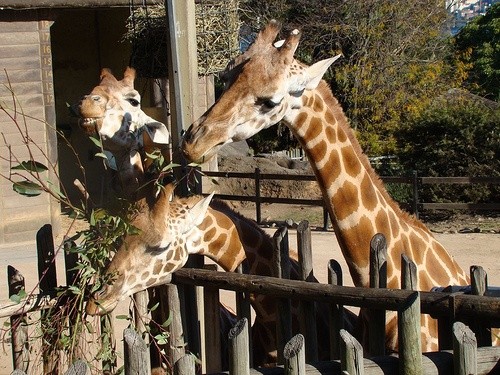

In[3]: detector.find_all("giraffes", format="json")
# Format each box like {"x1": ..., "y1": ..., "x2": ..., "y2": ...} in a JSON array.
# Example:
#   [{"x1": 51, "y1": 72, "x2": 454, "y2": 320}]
[
  {"x1": 76, "y1": 66, "x2": 238, "y2": 374},
  {"x1": 85, "y1": 183, "x2": 368, "y2": 373},
  {"x1": 181, "y1": 19, "x2": 499, "y2": 355}
]
